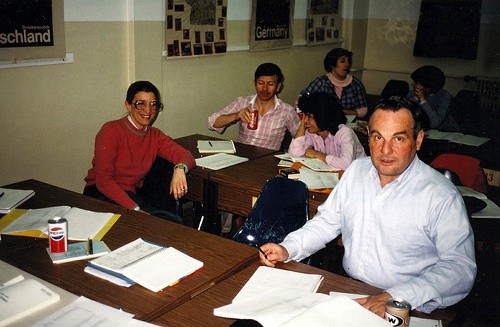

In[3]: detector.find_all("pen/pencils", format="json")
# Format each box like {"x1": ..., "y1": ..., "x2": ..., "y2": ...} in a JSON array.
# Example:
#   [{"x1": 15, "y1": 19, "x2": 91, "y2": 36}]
[
  {"x1": 86, "y1": 237, "x2": 93, "y2": 255},
  {"x1": 209, "y1": 141, "x2": 212, "y2": 146},
  {"x1": 0, "y1": 192, "x2": 5, "y2": 197},
  {"x1": 255, "y1": 244, "x2": 268, "y2": 259}
]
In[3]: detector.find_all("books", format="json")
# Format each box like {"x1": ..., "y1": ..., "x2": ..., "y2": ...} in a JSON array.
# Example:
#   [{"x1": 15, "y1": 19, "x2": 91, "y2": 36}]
[
  {"x1": 214, "y1": 264, "x2": 444, "y2": 327},
  {"x1": 0, "y1": 187, "x2": 36, "y2": 214},
  {"x1": 194, "y1": 152, "x2": 248, "y2": 170},
  {"x1": 455, "y1": 185, "x2": 500, "y2": 219},
  {"x1": 273, "y1": 151, "x2": 339, "y2": 188},
  {"x1": 196, "y1": 139, "x2": 236, "y2": 155},
  {"x1": 0, "y1": 206, "x2": 121, "y2": 242},
  {"x1": 0, "y1": 274, "x2": 61, "y2": 327},
  {"x1": 427, "y1": 131, "x2": 489, "y2": 147},
  {"x1": 46, "y1": 242, "x2": 205, "y2": 292}
]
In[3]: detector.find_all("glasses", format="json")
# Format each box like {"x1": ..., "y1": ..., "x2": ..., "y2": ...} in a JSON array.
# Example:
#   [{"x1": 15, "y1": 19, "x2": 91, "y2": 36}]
[{"x1": 131, "y1": 100, "x2": 163, "y2": 112}]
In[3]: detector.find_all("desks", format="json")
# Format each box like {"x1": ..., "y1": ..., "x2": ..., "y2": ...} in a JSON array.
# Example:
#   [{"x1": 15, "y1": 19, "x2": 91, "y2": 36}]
[
  {"x1": 159, "y1": 134, "x2": 279, "y2": 226},
  {"x1": 149, "y1": 262, "x2": 454, "y2": 325},
  {"x1": 209, "y1": 151, "x2": 345, "y2": 240},
  {"x1": 1, "y1": 179, "x2": 261, "y2": 323},
  {"x1": 345, "y1": 116, "x2": 499, "y2": 172}
]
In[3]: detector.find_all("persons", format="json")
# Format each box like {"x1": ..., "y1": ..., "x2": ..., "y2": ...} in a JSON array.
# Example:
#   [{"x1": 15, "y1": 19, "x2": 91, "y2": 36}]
[
  {"x1": 286, "y1": 90, "x2": 364, "y2": 170},
  {"x1": 83, "y1": 81, "x2": 195, "y2": 216},
  {"x1": 206, "y1": 63, "x2": 308, "y2": 151},
  {"x1": 403, "y1": 65, "x2": 462, "y2": 135},
  {"x1": 297, "y1": 47, "x2": 368, "y2": 117},
  {"x1": 259, "y1": 90, "x2": 476, "y2": 321}
]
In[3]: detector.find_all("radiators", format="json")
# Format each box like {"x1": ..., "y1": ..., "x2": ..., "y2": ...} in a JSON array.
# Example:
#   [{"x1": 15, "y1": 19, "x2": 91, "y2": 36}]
[{"x1": 463, "y1": 76, "x2": 500, "y2": 117}]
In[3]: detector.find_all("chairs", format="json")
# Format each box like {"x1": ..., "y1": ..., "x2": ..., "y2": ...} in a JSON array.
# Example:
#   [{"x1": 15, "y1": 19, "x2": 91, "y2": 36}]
[
  {"x1": 145, "y1": 160, "x2": 183, "y2": 224},
  {"x1": 427, "y1": 153, "x2": 490, "y2": 195},
  {"x1": 227, "y1": 176, "x2": 311, "y2": 267},
  {"x1": 371, "y1": 78, "x2": 409, "y2": 113}
]
